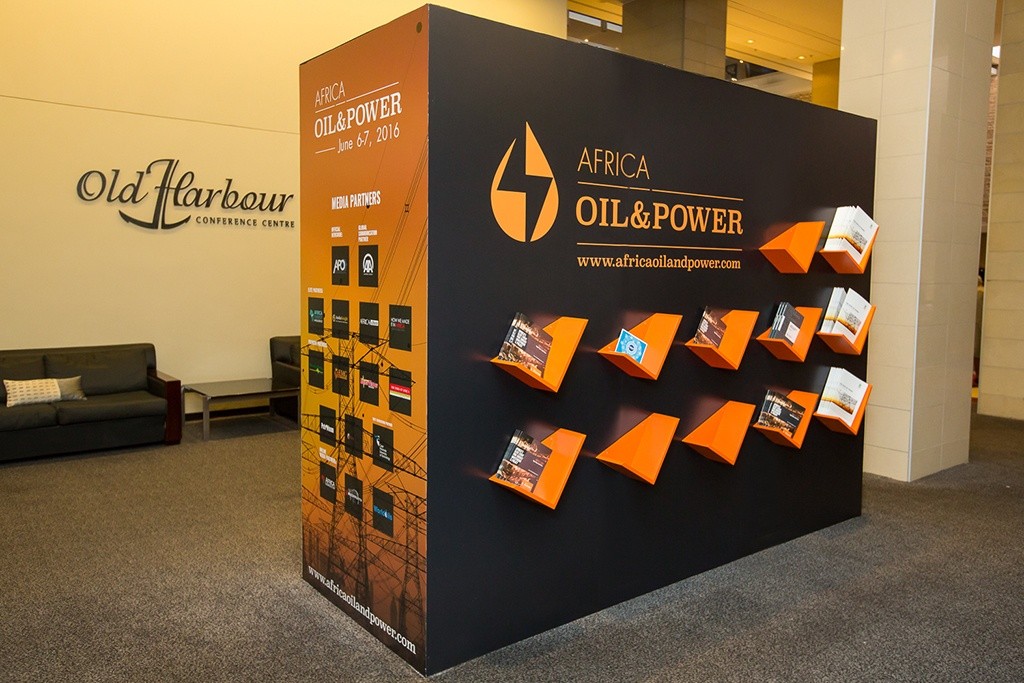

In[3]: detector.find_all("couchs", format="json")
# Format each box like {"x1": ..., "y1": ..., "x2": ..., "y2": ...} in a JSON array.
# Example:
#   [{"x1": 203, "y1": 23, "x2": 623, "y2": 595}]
[
  {"x1": 0, "y1": 343, "x2": 182, "y2": 464},
  {"x1": 270, "y1": 336, "x2": 301, "y2": 424}
]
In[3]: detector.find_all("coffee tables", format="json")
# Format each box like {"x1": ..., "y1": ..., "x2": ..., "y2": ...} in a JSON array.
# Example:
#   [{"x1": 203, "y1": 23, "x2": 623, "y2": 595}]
[{"x1": 181, "y1": 377, "x2": 302, "y2": 441}]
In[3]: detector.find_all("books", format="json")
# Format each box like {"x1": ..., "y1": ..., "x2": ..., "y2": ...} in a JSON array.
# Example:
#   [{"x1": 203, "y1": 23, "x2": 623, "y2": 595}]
[
  {"x1": 691, "y1": 306, "x2": 726, "y2": 352},
  {"x1": 759, "y1": 390, "x2": 807, "y2": 441},
  {"x1": 498, "y1": 311, "x2": 553, "y2": 379},
  {"x1": 820, "y1": 284, "x2": 873, "y2": 346},
  {"x1": 497, "y1": 429, "x2": 552, "y2": 494},
  {"x1": 823, "y1": 204, "x2": 878, "y2": 267},
  {"x1": 817, "y1": 366, "x2": 868, "y2": 428},
  {"x1": 766, "y1": 301, "x2": 804, "y2": 347}
]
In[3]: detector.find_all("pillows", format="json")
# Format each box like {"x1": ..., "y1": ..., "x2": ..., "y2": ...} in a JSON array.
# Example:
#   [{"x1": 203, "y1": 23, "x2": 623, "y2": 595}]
[{"x1": 3, "y1": 376, "x2": 86, "y2": 407}]
